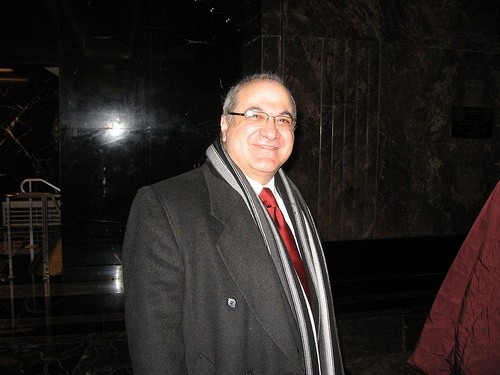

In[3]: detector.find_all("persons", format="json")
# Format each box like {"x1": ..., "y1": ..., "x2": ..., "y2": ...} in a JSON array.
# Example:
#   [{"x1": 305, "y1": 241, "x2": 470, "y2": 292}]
[{"x1": 123, "y1": 71, "x2": 346, "y2": 374}]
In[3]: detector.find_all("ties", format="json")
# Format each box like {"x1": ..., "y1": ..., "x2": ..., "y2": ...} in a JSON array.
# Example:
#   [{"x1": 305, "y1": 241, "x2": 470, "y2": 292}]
[{"x1": 259, "y1": 188, "x2": 312, "y2": 304}]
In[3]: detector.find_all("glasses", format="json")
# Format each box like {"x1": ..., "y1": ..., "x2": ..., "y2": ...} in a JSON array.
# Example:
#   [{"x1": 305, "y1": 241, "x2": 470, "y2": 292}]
[{"x1": 226, "y1": 110, "x2": 297, "y2": 130}]
